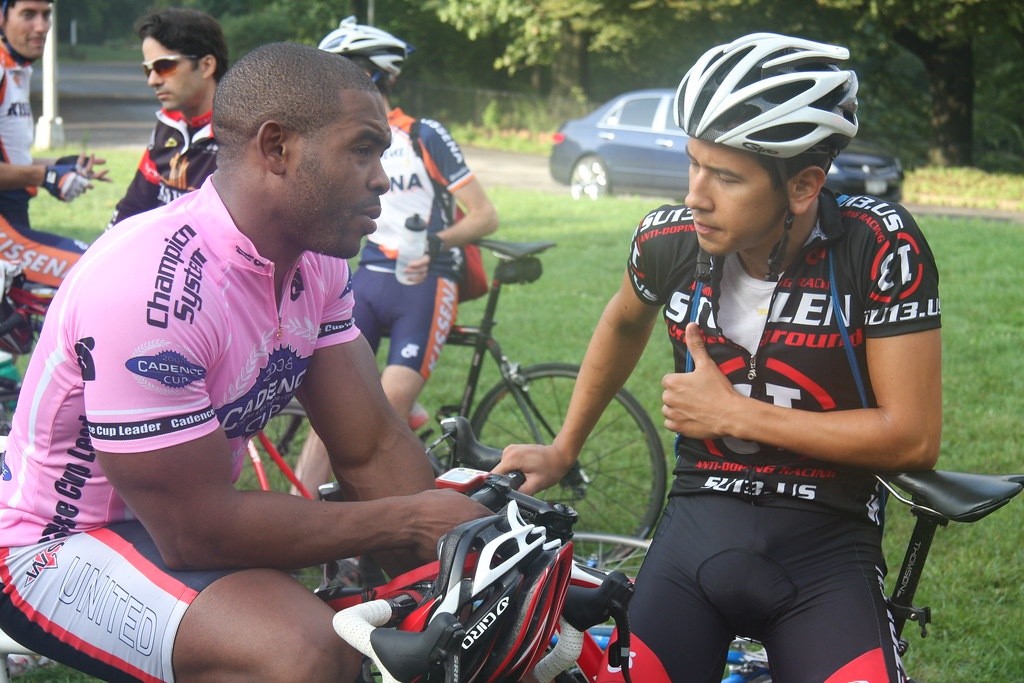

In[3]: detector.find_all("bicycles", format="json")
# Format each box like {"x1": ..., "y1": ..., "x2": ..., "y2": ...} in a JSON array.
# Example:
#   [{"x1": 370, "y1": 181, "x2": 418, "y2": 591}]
[
  {"x1": 1, "y1": 403, "x2": 1024, "y2": 683},
  {"x1": 1, "y1": 246, "x2": 58, "y2": 416},
  {"x1": 237, "y1": 238, "x2": 669, "y2": 577}
]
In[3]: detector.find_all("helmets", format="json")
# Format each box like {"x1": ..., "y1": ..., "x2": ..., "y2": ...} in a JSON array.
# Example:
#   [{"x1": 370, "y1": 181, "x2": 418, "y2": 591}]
[
  {"x1": 318, "y1": 16, "x2": 414, "y2": 82},
  {"x1": 672, "y1": 31, "x2": 859, "y2": 157}
]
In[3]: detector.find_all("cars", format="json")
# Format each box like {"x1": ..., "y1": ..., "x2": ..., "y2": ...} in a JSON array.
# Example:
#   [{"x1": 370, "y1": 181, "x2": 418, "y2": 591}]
[{"x1": 548, "y1": 87, "x2": 905, "y2": 205}]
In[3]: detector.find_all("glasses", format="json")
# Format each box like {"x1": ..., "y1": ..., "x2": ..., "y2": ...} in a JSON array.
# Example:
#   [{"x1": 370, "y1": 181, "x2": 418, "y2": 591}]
[{"x1": 142, "y1": 55, "x2": 197, "y2": 78}]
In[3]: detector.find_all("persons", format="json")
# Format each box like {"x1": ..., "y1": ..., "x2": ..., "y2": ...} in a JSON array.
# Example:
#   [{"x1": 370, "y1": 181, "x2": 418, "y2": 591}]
[
  {"x1": 0, "y1": 40, "x2": 503, "y2": 683},
  {"x1": 489, "y1": 32, "x2": 943, "y2": 683},
  {"x1": 291, "y1": 15, "x2": 498, "y2": 502},
  {"x1": 102, "y1": 9, "x2": 228, "y2": 237},
  {"x1": 0, "y1": 0, "x2": 113, "y2": 293}
]
